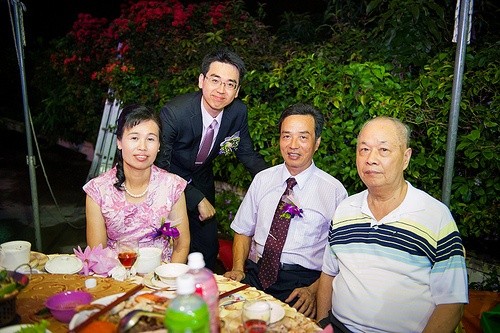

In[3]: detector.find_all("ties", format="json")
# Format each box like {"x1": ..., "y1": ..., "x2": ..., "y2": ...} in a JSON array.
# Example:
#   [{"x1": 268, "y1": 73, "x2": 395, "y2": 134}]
[
  {"x1": 257, "y1": 178, "x2": 297, "y2": 290},
  {"x1": 194, "y1": 120, "x2": 218, "y2": 168}
]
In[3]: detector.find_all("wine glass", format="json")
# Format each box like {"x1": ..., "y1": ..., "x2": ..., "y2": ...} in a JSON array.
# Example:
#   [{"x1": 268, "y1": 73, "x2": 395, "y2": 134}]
[{"x1": 116, "y1": 237, "x2": 140, "y2": 279}]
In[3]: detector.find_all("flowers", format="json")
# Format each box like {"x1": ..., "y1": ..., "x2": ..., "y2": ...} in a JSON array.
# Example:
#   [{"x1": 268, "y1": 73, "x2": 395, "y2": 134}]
[
  {"x1": 151, "y1": 217, "x2": 180, "y2": 247},
  {"x1": 279, "y1": 195, "x2": 304, "y2": 219},
  {"x1": 220, "y1": 131, "x2": 241, "y2": 160}
]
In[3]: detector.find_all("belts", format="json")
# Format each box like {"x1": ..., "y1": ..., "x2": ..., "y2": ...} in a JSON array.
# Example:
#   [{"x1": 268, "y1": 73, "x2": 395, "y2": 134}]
[{"x1": 280, "y1": 263, "x2": 311, "y2": 273}]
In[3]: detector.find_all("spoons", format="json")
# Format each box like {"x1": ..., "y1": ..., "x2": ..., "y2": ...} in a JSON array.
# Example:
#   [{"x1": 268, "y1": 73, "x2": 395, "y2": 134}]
[{"x1": 118, "y1": 309, "x2": 165, "y2": 333}]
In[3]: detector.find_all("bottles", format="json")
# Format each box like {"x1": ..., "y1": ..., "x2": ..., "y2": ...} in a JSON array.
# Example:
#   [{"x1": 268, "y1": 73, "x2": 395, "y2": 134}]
[
  {"x1": 187, "y1": 252, "x2": 220, "y2": 333},
  {"x1": 163, "y1": 273, "x2": 211, "y2": 333}
]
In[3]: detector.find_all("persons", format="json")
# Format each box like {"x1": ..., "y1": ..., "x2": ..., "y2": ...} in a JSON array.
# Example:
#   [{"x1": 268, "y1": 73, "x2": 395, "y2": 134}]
[
  {"x1": 81, "y1": 104, "x2": 191, "y2": 266},
  {"x1": 222, "y1": 103, "x2": 349, "y2": 325},
  {"x1": 155, "y1": 48, "x2": 270, "y2": 276},
  {"x1": 316, "y1": 116, "x2": 470, "y2": 333}
]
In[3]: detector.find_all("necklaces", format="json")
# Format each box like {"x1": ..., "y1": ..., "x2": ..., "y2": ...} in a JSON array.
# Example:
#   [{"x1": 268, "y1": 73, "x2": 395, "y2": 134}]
[{"x1": 121, "y1": 180, "x2": 151, "y2": 198}]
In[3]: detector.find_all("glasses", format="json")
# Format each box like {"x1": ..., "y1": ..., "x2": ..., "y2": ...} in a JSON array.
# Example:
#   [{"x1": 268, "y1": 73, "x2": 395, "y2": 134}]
[{"x1": 204, "y1": 75, "x2": 239, "y2": 92}]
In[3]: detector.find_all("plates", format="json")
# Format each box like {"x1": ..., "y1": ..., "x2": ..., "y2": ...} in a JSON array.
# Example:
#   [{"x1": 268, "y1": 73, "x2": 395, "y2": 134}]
[
  {"x1": 68, "y1": 290, "x2": 176, "y2": 332},
  {"x1": 142, "y1": 271, "x2": 177, "y2": 291},
  {"x1": 246, "y1": 300, "x2": 285, "y2": 324},
  {"x1": 29, "y1": 251, "x2": 49, "y2": 271},
  {"x1": 44, "y1": 255, "x2": 84, "y2": 274}
]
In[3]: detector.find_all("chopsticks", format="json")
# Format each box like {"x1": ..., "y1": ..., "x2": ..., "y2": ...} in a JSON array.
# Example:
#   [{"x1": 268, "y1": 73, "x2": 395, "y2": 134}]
[{"x1": 219, "y1": 284, "x2": 250, "y2": 299}]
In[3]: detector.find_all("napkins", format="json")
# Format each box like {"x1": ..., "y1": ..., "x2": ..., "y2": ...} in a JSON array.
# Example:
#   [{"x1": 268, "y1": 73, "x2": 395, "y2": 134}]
[{"x1": 74, "y1": 243, "x2": 117, "y2": 274}]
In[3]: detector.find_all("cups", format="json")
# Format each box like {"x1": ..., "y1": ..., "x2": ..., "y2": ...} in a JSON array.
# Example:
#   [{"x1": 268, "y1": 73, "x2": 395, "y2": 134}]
[
  {"x1": 0, "y1": 240, "x2": 31, "y2": 273},
  {"x1": 242, "y1": 300, "x2": 271, "y2": 333}
]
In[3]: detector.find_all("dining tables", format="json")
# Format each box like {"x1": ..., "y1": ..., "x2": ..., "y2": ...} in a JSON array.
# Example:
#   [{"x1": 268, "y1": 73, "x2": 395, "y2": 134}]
[{"x1": 0, "y1": 254, "x2": 324, "y2": 333}]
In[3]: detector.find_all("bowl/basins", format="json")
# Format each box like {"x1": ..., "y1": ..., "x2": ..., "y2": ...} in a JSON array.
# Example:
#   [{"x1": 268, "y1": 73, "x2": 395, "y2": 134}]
[
  {"x1": 44, "y1": 291, "x2": 93, "y2": 324},
  {"x1": 0, "y1": 323, "x2": 51, "y2": 333},
  {"x1": 154, "y1": 263, "x2": 190, "y2": 285},
  {"x1": 136, "y1": 247, "x2": 163, "y2": 275}
]
